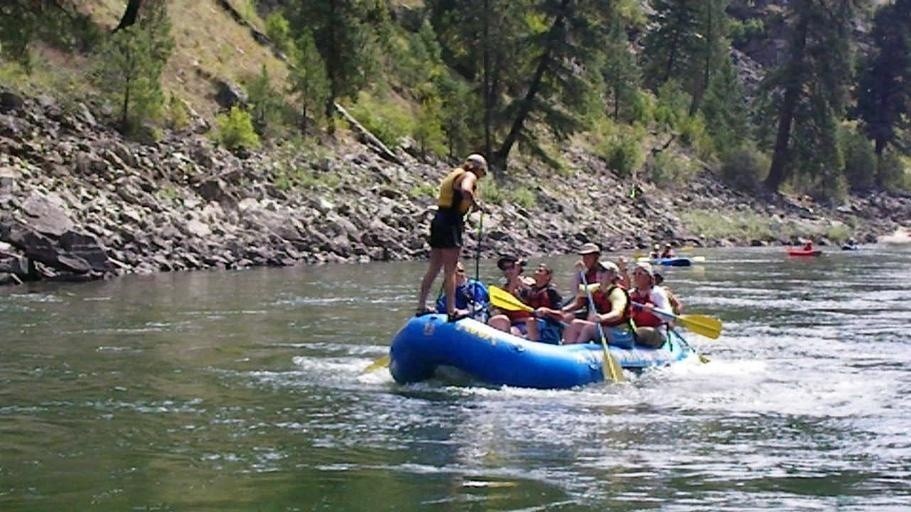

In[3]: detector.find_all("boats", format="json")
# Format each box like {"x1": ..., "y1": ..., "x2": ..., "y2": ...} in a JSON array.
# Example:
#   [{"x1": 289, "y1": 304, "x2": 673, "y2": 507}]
[
  {"x1": 788, "y1": 250, "x2": 822, "y2": 257},
  {"x1": 638, "y1": 257, "x2": 691, "y2": 267},
  {"x1": 388, "y1": 313, "x2": 685, "y2": 389}
]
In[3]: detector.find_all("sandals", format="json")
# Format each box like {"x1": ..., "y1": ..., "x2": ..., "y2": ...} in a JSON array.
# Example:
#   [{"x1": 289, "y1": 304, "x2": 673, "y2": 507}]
[
  {"x1": 448, "y1": 309, "x2": 469, "y2": 322},
  {"x1": 415, "y1": 306, "x2": 434, "y2": 316}
]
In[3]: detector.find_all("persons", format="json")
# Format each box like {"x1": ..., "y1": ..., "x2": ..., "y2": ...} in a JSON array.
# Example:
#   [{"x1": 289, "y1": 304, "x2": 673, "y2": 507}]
[
  {"x1": 436, "y1": 239, "x2": 687, "y2": 350},
  {"x1": 414, "y1": 149, "x2": 491, "y2": 324},
  {"x1": 801, "y1": 239, "x2": 816, "y2": 256}
]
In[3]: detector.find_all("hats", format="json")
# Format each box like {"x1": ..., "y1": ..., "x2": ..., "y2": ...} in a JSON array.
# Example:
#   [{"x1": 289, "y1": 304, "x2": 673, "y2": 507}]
[
  {"x1": 465, "y1": 154, "x2": 488, "y2": 175},
  {"x1": 497, "y1": 243, "x2": 671, "y2": 279}
]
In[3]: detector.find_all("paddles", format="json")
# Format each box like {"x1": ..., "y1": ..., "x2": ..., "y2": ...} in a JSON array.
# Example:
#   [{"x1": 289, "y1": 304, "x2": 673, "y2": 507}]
[
  {"x1": 580, "y1": 272, "x2": 626, "y2": 381},
  {"x1": 362, "y1": 353, "x2": 392, "y2": 373},
  {"x1": 668, "y1": 325, "x2": 722, "y2": 369},
  {"x1": 488, "y1": 285, "x2": 568, "y2": 328},
  {"x1": 628, "y1": 293, "x2": 723, "y2": 341}
]
